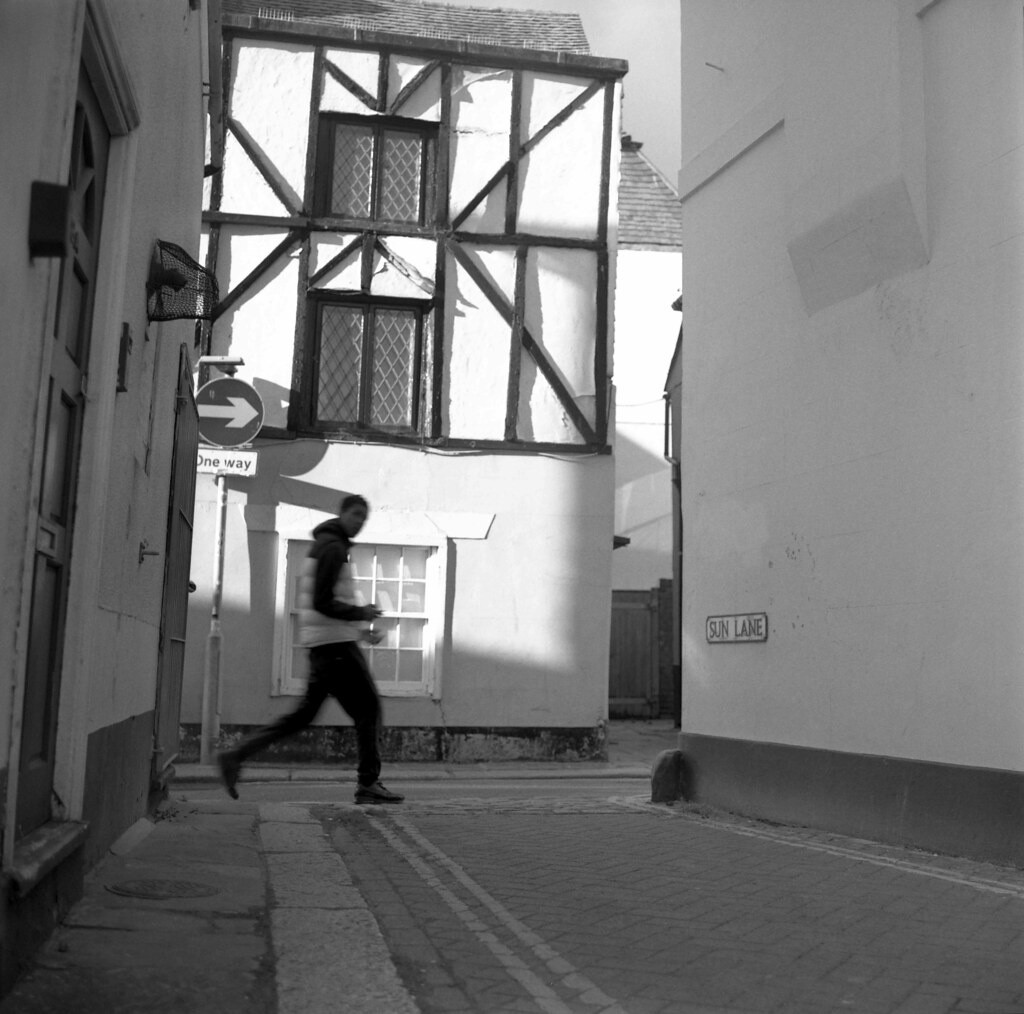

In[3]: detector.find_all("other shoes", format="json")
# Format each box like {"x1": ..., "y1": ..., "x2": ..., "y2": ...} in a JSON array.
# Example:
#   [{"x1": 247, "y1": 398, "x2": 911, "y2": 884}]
[
  {"x1": 214, "y1": 753, "x2": 240, "y2": 798},
  {"x1": 354, "y1": 779, "x2": 407, "y2": 803}
]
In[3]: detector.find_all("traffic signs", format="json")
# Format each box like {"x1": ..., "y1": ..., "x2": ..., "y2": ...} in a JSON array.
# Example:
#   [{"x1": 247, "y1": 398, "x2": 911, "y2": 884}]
[{"x1": 195, "y1": 446, "x2": 259, "y2": 478}]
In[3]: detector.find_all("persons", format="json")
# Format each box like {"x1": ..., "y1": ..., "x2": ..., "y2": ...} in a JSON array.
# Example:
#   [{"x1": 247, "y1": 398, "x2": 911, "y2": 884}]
[{"x1": 217, "y1": 494, "x2": 406, "y2": 804}]
